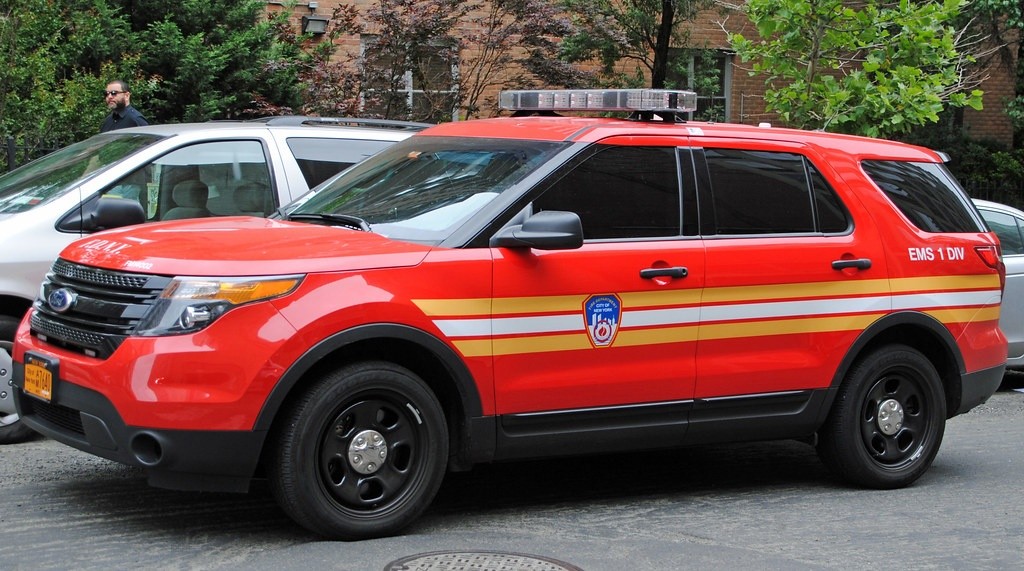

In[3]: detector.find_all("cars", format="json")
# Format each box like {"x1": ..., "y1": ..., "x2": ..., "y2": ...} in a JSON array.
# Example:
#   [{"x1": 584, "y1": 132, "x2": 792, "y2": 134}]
[
  {"x1": 11, "y1": 88, "x2": 1009, "y2": 537},
  {"x1": 968, "y1": 199, "x2": 1024, "y2": 368}
]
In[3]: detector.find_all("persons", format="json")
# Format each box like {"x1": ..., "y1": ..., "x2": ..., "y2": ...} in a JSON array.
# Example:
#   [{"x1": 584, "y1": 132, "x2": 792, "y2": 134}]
[{"x1": 80, "y1": 80, "x2": 149, "y2": 202}]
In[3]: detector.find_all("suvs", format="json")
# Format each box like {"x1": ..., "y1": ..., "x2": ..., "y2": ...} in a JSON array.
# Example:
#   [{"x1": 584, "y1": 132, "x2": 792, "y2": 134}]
[{"x1": 0, "y1": 116, "x2": 532, "y2": 447}]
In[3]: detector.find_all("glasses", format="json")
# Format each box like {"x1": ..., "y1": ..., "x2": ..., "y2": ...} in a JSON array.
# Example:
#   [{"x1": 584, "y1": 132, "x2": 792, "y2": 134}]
[{"x1": 103, "y1": 91, "x2": 127, "y2": 97}]
